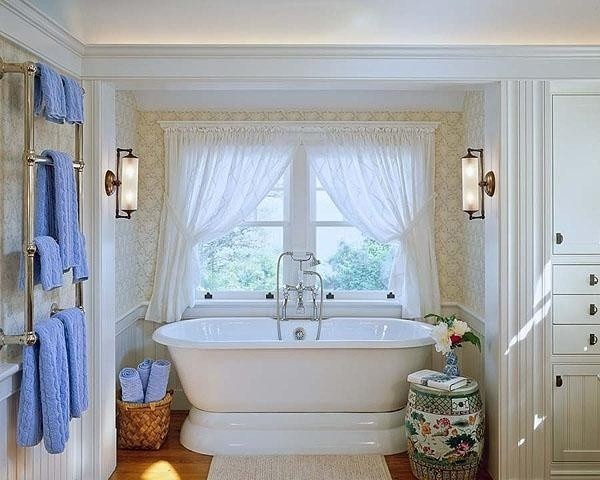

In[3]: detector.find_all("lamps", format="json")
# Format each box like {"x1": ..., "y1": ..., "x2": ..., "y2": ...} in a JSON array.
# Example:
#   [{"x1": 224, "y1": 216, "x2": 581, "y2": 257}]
[
  {"x1": 106, "y1": 145, "x2": 139, "y2": 220},
  {"x1": 459, "y1": 146, "x2": 496, "y2": 223}
]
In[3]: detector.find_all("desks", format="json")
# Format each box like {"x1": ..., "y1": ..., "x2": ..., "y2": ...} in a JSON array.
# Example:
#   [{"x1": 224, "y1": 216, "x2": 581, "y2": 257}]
[{"x1": 403, "y1": 375, "x2": 485, "y2": 480}]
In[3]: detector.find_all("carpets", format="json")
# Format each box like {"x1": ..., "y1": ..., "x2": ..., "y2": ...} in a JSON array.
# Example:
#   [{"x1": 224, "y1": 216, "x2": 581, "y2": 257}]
[{"x1": 204, "y1": 454, "x2": 393, "y2": 479}]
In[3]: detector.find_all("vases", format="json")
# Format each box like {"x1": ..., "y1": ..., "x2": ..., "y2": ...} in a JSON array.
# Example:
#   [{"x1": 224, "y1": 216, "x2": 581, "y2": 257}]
[{"x1": 443, "y1": 349, "x2": 460, "y2": 377}]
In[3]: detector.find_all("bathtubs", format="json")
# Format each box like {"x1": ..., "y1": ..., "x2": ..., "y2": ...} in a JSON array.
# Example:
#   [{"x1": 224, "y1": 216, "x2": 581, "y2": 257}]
[{"x1": 152, "y1": 318, "x2": 438, "y2": 412}]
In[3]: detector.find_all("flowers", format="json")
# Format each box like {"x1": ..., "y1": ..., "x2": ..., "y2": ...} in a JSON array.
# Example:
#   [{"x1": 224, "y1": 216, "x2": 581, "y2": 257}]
[{"x1": 422, "y1": 311, "x2": 480, "y2": 357}]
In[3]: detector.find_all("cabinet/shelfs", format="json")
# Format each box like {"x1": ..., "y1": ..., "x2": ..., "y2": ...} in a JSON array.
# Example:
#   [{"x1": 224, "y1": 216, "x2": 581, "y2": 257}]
[{"x1": 542, "y1": 79, "x2": 600, "y2": 480}]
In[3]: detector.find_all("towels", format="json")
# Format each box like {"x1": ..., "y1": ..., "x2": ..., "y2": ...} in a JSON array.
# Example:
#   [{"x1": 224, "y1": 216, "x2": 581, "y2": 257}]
[
  {"x1": 33, "y1": 63, "x2": 66, "y2": 123},
  {"x1": 16, "y1": 317, "x2": 72, "y2": 456},
  {"x1": 72, "y1": 233, "x2": 89, "y2": 285},
  {"x1": 37, "y1": 149, "x2": 83, "y2": 272},
  {"x1": 118, "y1": 359, "x2": 172, "y2": 408},
  {"x1": 61, "y1": 74, "x2": 86, "y2": 126},
  {"x1": 52, "y1": 306, "x2": 89, "y2": 420},
  {"x1": 19, "y1": 236, "x2": 64, "y2": 292}
]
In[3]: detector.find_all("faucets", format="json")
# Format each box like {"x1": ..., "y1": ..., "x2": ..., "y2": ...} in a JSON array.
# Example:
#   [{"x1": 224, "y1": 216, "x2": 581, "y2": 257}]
[{"x1": 296, "y1": 302, "x2": 306, "y2": 315}]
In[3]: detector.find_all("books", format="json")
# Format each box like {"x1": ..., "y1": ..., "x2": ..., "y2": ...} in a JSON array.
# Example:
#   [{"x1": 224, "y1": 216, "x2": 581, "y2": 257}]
[{"x1": 407, "y1": 369, "x2": 468, "y2": 391}]
